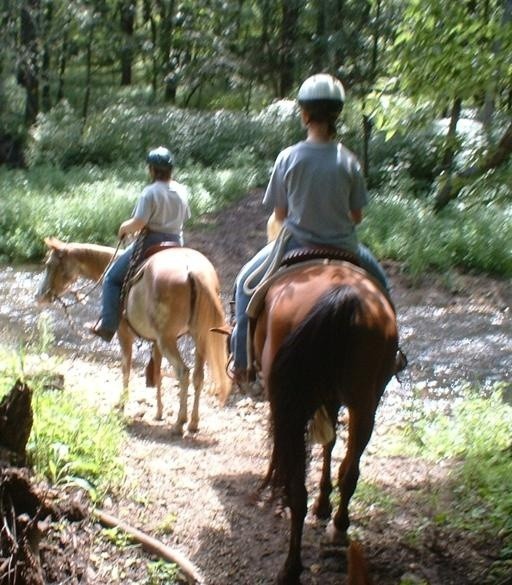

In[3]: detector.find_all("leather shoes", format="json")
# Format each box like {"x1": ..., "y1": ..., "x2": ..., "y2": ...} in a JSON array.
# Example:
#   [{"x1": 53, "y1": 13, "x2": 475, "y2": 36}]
[
  {"x1": 83, "y1": 321, "x2": 114, "y2": 342},
  {"x1": 228, "y1": 361, "x2": 256, "y2": 382}
]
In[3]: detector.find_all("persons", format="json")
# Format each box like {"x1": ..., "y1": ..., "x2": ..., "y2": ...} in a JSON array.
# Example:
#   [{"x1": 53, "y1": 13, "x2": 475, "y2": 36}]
[
  {"x1": 223, "y1": 71, "x2": 409, "y2": 389},
  {"x1": 85, "y1": 145, "x2": 193, "y2": 343}
]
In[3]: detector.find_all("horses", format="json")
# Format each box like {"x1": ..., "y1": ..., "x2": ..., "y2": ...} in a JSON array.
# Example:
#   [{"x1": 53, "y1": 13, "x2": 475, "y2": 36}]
[
  {"x1": 33, "y1": 234, "x2": 235, "y2": 440},
  {"x1": 248, "y1": 206, "x2": 405, "y2": 585}
]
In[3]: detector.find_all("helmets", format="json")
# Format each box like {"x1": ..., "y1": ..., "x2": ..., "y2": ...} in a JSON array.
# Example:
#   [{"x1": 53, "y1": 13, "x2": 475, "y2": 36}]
[
  {"x1": 146, "y1": 147, "x2": 173, "y2": 168},
  {"x1": 297, "y1": 74, "x2": 346, "y2": 103}
]
